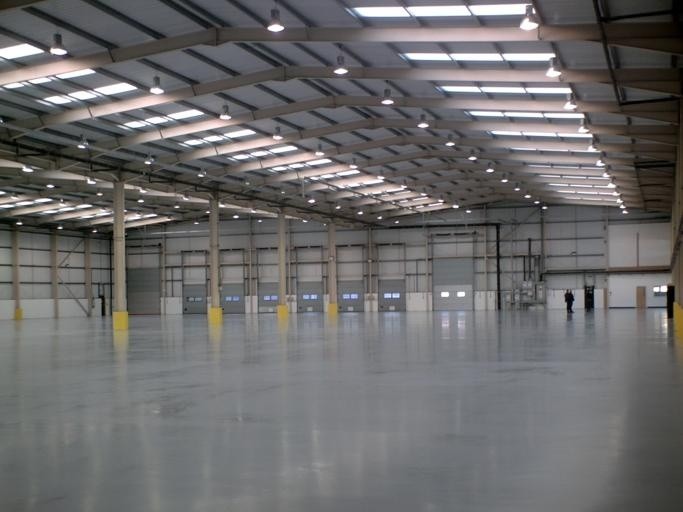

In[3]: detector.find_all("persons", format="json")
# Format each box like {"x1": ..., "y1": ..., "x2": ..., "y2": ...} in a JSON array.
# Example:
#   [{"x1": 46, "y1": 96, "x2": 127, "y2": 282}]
[
  {"x1": 585, "y1": 289, "x2": 593, "y2": 311},
  {"x1": 569, "y1": 290, "x2": 574, "y2": 312},
  {"x1": 564, "y1": 289, "x2": 570, "y2": 313}
]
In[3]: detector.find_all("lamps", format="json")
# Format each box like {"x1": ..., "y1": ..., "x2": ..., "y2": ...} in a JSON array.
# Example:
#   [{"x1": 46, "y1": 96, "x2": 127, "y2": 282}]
[
  {"x1": 332, "y1": 55, "x2": 350, "y2": 76},
  {"x1": 416, "y1": 113, "x2": 430, "y2": 129},
  {"x1": 380, "y1": 88, "x2": 395, "y2": 106},
  {"x1": 149, "y1": 76, "x2": 164, "y2": 95},
  {"x1": 468, "y1": 149, "x2": 479, "y2": 161},
  {"x1": 444, "y1": 134, "x2": 455, "y2": 148},
  {"x1": 219, "y1": 103, "x2": 233, "y2": 121},
  {"x1": 16, "y1": 155, "x2": 631, "y2": 234},
  {"x1": 144, "y1": 151, "x2": 154, "y2": 164},
  {"x1": 316, "y1": 143, "x2": 326, "y2": 156},
  {"x1": 578, "y1": 118, "x2": 591, "y2": 134},
  {"x1": 589, "y1": 138, "x2": 600, "y2": 153},
  {"x1": 266, "y1": 8, "x2": 285, "y2": 33},
  {"x1": 518, "y1": 5, "x2": 542, "y2": 31},
  {"x1": 77, "y1": 134, "x2": 89, "y2": 150},
  {"x1": 48, "y1": 34, "x2": 69, "y2": 57},
  {"x1": 545, "y1": 57, "x2": 562, "y2": 78},
  {"x1": 272, "y1": 126, "x2": 284, "y2": 141},
  {"x1": 564, "y1": 93, "x2": 578, "y2": 111}
]
